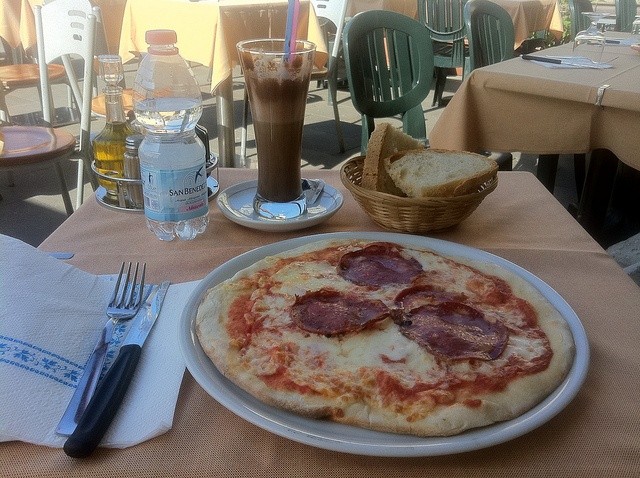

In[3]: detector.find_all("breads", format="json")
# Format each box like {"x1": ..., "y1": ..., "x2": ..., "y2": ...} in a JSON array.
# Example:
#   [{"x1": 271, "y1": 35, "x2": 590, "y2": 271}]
[
  {"x1": 361, "y1": 122, "x2": 426, "y2": 192},
  {"x1": 383, "y1": 148, "x2": 499, "y2": 198}
]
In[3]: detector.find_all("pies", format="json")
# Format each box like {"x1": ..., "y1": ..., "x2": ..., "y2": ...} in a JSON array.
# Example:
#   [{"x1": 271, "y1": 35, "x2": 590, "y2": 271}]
[{"x1": 193, "y1": 237, "x2": 576, "y2": 436}]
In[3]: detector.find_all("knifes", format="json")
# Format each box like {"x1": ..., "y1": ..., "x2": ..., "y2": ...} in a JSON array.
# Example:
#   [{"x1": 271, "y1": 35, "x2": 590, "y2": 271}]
[
  {"x1": 521, "y1": 54, "x2": 600, "y2": 69},
  {"x1": 63, "y1": 279, "x2": 171, "y2": 459}
]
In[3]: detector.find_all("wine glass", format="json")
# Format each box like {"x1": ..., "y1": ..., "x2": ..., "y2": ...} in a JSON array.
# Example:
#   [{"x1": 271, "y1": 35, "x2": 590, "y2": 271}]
[
  {"x1": 573, "y1": 12, "x2": 611, "y2": 68},
  {"x1": 632, "y1": 15, "x2": 640, "y2": 45}
]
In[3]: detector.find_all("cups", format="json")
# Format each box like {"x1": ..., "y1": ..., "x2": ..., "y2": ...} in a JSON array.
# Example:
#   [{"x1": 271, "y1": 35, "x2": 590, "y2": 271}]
[{"x1": 235, "y1": 37, "x2": 317, "y2": 222}]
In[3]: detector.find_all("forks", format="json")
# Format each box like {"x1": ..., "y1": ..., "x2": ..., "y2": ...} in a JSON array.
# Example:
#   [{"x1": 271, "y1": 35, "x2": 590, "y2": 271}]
[{"x1": 54, "y1": 261, "x2": 146, "y2": 438}]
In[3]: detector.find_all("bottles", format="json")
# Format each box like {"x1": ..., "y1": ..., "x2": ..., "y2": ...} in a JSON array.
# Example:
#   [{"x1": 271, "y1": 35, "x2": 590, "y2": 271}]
[
  {"x1": 92, "y1": 53, "x2": 142, "y2": 202},
  {"x1": 132, "y1": 29, "x2": 210, "y2": 243},
  {"x1": 123, "y1": 133, "x2": 146, "y2": 209}
]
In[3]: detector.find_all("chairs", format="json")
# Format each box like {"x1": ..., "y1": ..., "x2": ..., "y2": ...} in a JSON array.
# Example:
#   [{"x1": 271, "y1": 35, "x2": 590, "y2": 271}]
[
  {"x1": 41, "y1": 0, "x2": 144, "y2": 211},
  {"x1": 569, "y1": 0, "x2": 594, "y2": 44},
  {"x1": 413, "y1": 0, "x2": 471, "y2": 108},
  {"x1": 239, "y1": 0, "x2": 347, "y2": 166},
  {"x1": 0, "y1": 0, "x2": 97, "y2": 215},
  {"x1": 614, "y1": 0, "x2": 637, "y2": 32},
  {"x1": 462, "y1": 1, "x2": 586, "y2": 204},
  {"x1": 342, "y1": 9, "x2": 435, "y2": 156},
  {"x1": 0, "y1": 0, "x2": 82, "y2": 126}
]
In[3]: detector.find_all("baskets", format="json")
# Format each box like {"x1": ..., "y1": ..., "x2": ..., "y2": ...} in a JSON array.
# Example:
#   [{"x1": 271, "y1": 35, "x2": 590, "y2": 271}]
[{"x1": 340, "y1": 156, "x2": 498, "y2": 235}]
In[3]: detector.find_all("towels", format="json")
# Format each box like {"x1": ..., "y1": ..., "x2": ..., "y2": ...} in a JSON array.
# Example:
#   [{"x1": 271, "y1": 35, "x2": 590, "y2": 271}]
[{"x1": 0, "y1": 232, "x2": 204, "y2": 449}]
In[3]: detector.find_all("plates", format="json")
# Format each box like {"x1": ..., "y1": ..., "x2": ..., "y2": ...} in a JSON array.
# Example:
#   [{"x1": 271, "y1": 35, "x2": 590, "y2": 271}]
[
  {"x1": 216, "y1": 179, "x2": 345, "y2": 233},
  {"x1": 177, "y1": 230, "x2": 592, "y2": 458}
]
